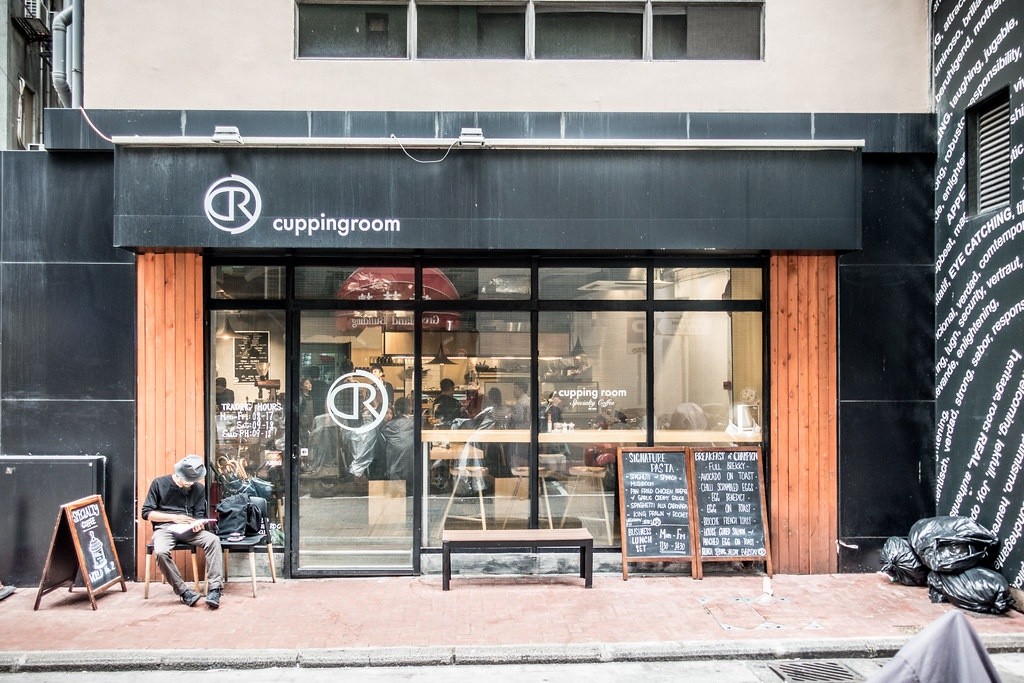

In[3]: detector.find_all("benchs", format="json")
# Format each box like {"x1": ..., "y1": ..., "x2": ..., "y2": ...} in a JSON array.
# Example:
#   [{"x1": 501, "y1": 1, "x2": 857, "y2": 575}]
[{"x1": 441, "y1": 527, "x2": 594, "y2": 591}]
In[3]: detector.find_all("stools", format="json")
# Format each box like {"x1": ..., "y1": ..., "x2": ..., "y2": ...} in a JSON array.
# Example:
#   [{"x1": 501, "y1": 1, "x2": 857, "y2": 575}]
[
  {"x1": 560, "y1": 466, "x2": 612, "y2": 545},
  {"x1": 436, "y1": 466, "x2": 489, "y2": 539},
  {"x1": 502, "y1": 466, "x2": 552, "y2": 530}
]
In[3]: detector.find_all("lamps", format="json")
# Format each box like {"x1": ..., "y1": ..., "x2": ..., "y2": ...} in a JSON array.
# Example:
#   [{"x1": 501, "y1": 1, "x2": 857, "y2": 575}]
[
  {"x1": 458, "y1": 127, "x2": 485, "y2": 145},
  {"x1": 211, "y1": 125, "x2": 243, "y2": 144},
  {"x1": 424, "y1": 332, "x2": 456, "y2": 366},
  {"x1": 234, "y1": 311, "x2": 263, "y2": 358},
  {"x1": 570, "y1": 312, "x2": 588, "y2": 359}
]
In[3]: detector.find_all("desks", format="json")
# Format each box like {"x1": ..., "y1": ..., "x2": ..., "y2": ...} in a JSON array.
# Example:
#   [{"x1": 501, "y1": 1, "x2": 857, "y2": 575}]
[
  {"x1": 429, "y1": 442, "x2": 484, "y2": 460},
  {"x1": 418, "y1": 430, "x2": 763, "y2": 547}
]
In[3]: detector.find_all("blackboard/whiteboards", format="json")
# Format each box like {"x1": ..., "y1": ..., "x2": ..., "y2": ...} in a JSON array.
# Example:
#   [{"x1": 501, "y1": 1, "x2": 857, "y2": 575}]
[
  {"x1": 690, "y1": 446, "x2": 772, "y2": 561},
  {"x1": 39, "y1": 494, "x2": 124, "y2": 597},
  {"x1": 617, "y1": 446, "x2": 697, "y2": 562}
]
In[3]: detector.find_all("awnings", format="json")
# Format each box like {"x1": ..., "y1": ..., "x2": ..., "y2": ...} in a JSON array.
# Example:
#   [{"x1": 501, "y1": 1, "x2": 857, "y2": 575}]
[{"x1": 334, "y1": 267, "x2": 459, "y2": 337}]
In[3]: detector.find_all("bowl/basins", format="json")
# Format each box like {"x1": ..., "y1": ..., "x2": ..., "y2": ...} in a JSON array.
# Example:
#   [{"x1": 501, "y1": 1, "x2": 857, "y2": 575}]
[{"x1": 442, "y1": 441, "x2": 449, "y2": 445}]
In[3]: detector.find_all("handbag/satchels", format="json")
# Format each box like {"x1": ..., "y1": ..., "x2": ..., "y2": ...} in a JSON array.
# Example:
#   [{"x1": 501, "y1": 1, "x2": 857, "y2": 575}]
[
  {"x1": 245, "y1": 503, "x2": 262, "y2": 535},
  {"x1": 214, "y1": 492, "x2": 246, "y2": 539}
]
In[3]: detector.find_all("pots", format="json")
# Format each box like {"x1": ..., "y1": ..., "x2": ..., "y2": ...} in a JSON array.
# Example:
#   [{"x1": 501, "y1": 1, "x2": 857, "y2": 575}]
[
  {"x1": 475, "y1": 361, "x2": 489, "y2": 371},
  {"x1": 490, "y1": 366, "x2": 505, "y2": 371}
]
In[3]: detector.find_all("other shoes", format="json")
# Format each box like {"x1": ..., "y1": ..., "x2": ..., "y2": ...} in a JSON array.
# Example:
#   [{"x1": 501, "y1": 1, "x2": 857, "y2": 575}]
[
  {"x1": 180, "y1": 589, "x2": 201, "y2": 606},
  {"x1": 205, "y1": 590, "x2": 221, "y2": 606}
]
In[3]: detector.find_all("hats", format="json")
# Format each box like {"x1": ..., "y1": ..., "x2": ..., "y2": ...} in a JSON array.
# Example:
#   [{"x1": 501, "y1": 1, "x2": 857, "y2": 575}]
[{"x1": 174, "y1": 455, "x2": 206, "y2": 481}]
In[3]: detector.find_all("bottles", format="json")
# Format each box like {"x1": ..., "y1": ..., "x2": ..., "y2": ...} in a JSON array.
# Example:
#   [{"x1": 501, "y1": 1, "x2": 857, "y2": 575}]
[
  {"x1": 548, "y1": 413, "x2": 552, "y2": 432},
  {"x1": 562, "y1": 421, "x2": 576, "y2": 432},
  {"x1": 370, "y1": 356, "x2": 392, "y2": 364}
]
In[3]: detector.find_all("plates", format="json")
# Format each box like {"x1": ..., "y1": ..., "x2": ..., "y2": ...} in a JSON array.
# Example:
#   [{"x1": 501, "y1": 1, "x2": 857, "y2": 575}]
[
  {"x1": 227, "y1": 537, "x2": 243, "y2": 541},
  {"x1": 433, "y1": 444, "x2": 439, "y2": 446},
  {"x1": 439, "y1": 445, "x2": 451, "y2": 447}
]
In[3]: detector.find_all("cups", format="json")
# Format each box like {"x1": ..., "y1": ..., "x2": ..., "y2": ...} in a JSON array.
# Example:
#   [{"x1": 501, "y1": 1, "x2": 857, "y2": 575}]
[
  {"x1": 538, "y1": 416, "x2": 548, "y2": 433},
  {"x1": 554, "y1": 422, "x2": 562, "y2": 432},
  {"x1": 230, "y1": 533, "x2": 240, "y2": 539}
]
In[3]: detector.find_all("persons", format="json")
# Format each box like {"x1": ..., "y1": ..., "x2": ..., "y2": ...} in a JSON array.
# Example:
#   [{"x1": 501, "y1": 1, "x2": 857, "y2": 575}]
[
  {"x1": 335, "y1": 359, "x2": 366, "y2": 428},
  {"x1": 433, "y1": 378, "x2": 469, "y2": 422},
  {"x1": 511, "y1": 381, "x2": 531, "y2": 429},
  {"x1": 309, "y1": 366, "x2": 329, "y2": 418},
  {"x1": 372, "y1": 402, "x2": 396, "y2": 430},
  {"x1": 216, "y1": 377, "x2": 235, "y2": 415},
  {"x1": 365, "y1": 366, "x2": 394, "y2": 421},
  {"x1": 472, "y1": 387, "x2": 512, "y2": 430},
  {"x1": 299, "y1": 378, "x2": 314, "y2": 448},
  {"x1": 142, "y1": 454, "x2": 225, "y2": 608},
  {"x1": 380, "y1": 397, "x2": 414, "y2": 497}
]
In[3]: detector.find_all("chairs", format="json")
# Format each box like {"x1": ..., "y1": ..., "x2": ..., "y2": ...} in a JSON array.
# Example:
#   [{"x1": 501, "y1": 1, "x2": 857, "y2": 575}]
[
  {"x1": 203, "y1": 496, "x2": 278, "y2": 598},
  {"x1": 144, "y1": 500, "x2": 210, "y2": 600}
]
